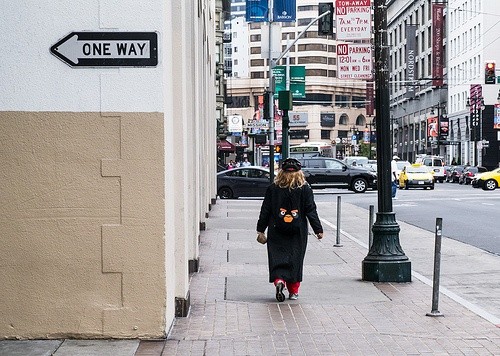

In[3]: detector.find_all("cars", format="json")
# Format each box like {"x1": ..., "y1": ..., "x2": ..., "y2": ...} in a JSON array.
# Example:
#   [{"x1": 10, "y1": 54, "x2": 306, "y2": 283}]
[
  {"x1": 443, "y1": 165, "x2": 466, "y2": 183},
  {"x1": 343, "y1": 156, "x2": 414, "y2": 186},
  {"x1": 471, "y1": 167, "x2": 500, "y2": 191},
  {"x1": 459, "y1": 166, "x2": 488, "y2": 185},
  {"x1": 217, "y1": 165, "x2": 278, "y2": 199},
  {"x1": 399, "y1": 164, "x2": 436, "y2": 190}
]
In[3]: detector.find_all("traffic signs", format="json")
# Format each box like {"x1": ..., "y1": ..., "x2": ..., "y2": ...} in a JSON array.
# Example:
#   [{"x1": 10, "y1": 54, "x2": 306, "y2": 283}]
[{"x1": 48, "y1": 30, "x2": 160, "y2": 69}]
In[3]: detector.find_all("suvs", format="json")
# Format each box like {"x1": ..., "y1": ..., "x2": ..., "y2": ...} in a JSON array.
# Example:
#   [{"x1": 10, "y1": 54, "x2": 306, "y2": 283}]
[{"x1": 296, "y1": 156, "x2": 378, "y2": 194}]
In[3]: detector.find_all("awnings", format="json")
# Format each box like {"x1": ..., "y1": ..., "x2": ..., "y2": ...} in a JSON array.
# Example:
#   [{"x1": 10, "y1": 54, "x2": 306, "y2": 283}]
[{"x1": 219, "y1": 139, "x2": 235, "y2": 153}]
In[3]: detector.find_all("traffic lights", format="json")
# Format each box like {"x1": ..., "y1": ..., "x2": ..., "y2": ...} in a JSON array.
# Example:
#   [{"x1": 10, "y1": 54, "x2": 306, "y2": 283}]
[
  {"x1": 484, "y1": 62, "x2": 495, "y2": 85},
  {"x1": 275, "y1": 144, "x2": 282, "y2": 152}
]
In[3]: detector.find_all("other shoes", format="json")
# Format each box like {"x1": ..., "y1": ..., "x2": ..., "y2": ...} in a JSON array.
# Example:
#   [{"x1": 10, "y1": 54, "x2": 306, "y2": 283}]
[
  {"x1": 289, "y1": 293, "x2": 298, "y2": 300},
  {"x1": 392, "y1": 197, "x2": 398, "y2": 200},
  {"x1": 276, "y1": 281, "x2": 285, "y2": 302}
]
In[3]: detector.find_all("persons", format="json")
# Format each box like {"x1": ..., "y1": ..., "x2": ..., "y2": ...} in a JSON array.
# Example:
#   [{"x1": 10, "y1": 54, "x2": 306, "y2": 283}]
[
  {"x1": 227, "y1": 159, "x2": 251, "y2": 176},
  {"x1": 256, "y1": 158, "x2": 323, "y2": 302},
  {"x1": 390, "y1": 156, "x2": 400, "y2": 197},
  {"x1": 427, "y1": 123, "x2": 438, "y2": 135}
]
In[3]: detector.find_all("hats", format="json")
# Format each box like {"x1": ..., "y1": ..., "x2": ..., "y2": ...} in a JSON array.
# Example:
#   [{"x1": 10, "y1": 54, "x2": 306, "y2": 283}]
[
  {"x1": 393, "y1": 156, "x2": 400, "y2": 159},
  {"x1": 282, "y1": 157, "x2": 301, "y2": 172}
]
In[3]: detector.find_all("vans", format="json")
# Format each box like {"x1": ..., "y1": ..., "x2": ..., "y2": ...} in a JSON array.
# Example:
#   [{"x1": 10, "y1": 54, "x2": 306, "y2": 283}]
[{"x1": 416, "y1": 154, "x2": 445, "y2": 185}]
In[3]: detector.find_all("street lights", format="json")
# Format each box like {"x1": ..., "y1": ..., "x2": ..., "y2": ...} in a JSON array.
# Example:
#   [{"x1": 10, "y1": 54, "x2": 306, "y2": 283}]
[
  {"x1": 430, "y1": 105, "x2": 447, "y2": 155},
  {"x1": 465, "y1": 95, "x2": 485, "y2": 168},
  {"x1": 365, "y1": 123, "x2": 376, "y2": 160},
  {"x1": 348, "y1": 127, "x2": 360, "y2": 157},
  {"x1": 390, "y1": 117, "x2": 399, "y2": 160}
]
